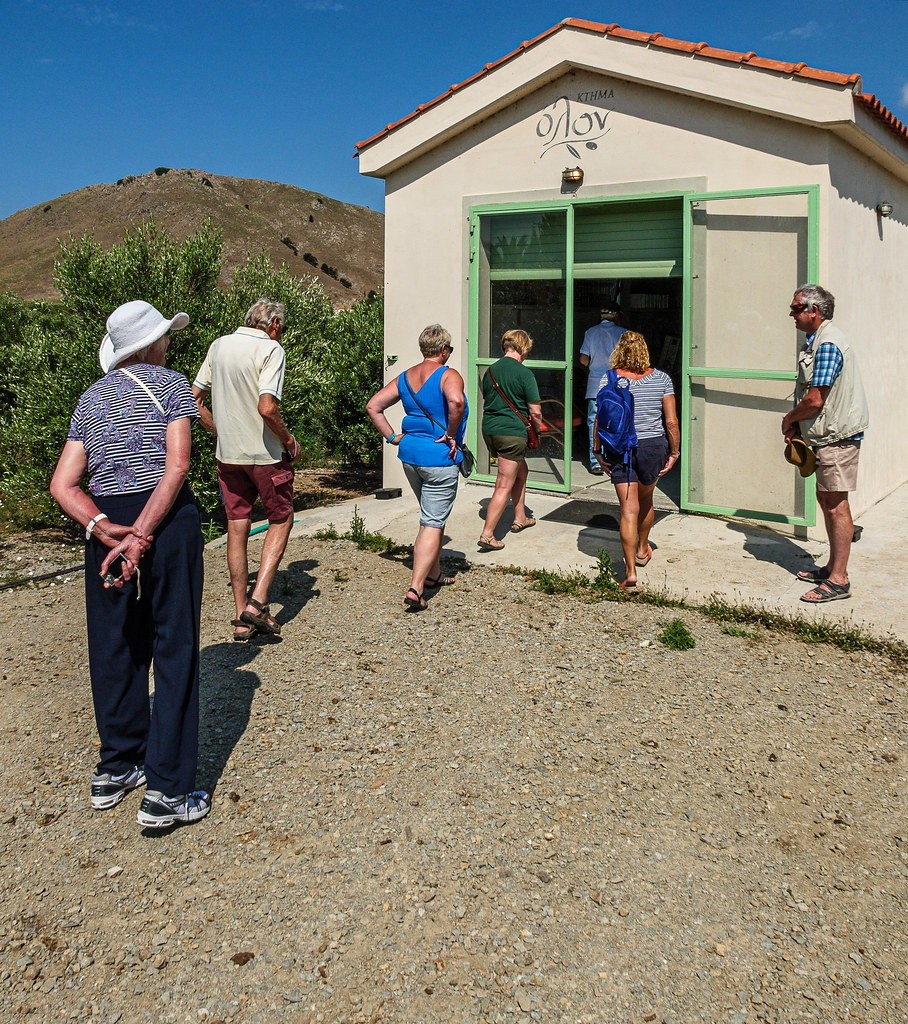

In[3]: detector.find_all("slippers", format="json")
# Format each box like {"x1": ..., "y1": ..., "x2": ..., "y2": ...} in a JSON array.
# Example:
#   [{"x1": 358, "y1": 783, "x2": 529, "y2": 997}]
[
  {"x1": 621, "y1": 581, "x2": 638, "y2": 593},
  {"x1": 635, "y1": 543, "x2": 652, "y2": 566}
]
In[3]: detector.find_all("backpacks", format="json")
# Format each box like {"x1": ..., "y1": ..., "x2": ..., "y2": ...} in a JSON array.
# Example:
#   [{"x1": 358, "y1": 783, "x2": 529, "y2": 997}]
[{"x1": 595, "y1": 369, "x2": 639, "y2": 487}]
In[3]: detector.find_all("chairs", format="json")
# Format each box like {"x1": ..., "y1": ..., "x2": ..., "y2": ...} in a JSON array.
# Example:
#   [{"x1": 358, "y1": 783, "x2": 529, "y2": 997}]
[{"x1": 539, "y1": 393, "x2": 586, "y2": 458}]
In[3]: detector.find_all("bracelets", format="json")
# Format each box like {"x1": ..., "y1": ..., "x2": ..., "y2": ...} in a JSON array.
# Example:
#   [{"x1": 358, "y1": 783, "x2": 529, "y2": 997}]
[
  {"x1": 445, "y1": 430, "x2": 457, "y2": 439},
  {"x1": 592, "y1": 447, "x2": 602, "y2": 454},
  {"x1": 386, "y1": 432, "x2": 396, "y2": 444},
  {"x1": 782, "y1": 416, "x2": 793, "y2": 429},
  {"x1": 86, "y1": 513, "x2": 109, "y2": 540},
  {"x1": 670, "y1": 451, "x2": 680, "y2": 458},
  {"x1": 286, "y1": 433, "x2": 297, "y2": 459}
]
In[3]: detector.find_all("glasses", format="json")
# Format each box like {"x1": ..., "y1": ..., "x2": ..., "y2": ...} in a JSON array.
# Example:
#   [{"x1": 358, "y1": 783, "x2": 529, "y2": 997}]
[
  {"x1": 281, "y1": 325, "x2": 289, "y2": 333},
  {"x1": 790, "y1": 303, "x2": 820, "y2": 314},
  {"x1": 446, "y1": 345, "x2": 453, "y2": 353}
]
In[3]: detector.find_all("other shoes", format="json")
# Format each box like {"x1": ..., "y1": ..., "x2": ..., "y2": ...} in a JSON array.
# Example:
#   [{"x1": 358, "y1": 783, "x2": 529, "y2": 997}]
[{"x1": 591, "y1": 468, "x2": 603, "y2": 474}]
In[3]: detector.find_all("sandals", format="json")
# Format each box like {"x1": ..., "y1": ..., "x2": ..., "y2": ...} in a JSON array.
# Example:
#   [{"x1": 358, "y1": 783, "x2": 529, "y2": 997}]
[
  {"x1": 425, "y1": 571, "x2": 455, "y2": 588},
  {"x1": 231, "y1": 620, "x2": 259, "y2": 640},
  {"x1": 797, "y1": 566, "x2": 848, "y2": 582},
  {"x1": 801, "y1": 580, "x2": 852, "y2": 603},
  {"x1": 404, "y1": 588, "x2": 428, "y2": 610},
  {"x1": 511, "y1": 518, "x2": 536, "y2": 533},
  {"x1": 477, "y1": 534, "x2": 505, "y2": 550},
  {"x1": 239, "y1": 598, "x2": 281, "y2": 635}
]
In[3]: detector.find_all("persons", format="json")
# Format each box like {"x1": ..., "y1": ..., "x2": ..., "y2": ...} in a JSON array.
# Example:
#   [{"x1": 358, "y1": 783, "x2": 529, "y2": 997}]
[
  {"x1": 191, "y1": 297, "x2": 301, "y2": 641},
  {"x1": 593, "y1": 331, "x2": 680, "y2": 585},
  {"x1": 51, "y1": 300, "x2": 212, "y2": 829},
  {"x1": 580, "y1": 301, "x2": 626, "y2": 474},
  {"x1": 781, "y1": 284, "x2": 870, "y2": 603},
  {"x1": 477, "y1": 329, "x2": 543, "y2": 549},
  {"x1": 367, "y1": 323, "x2": 470, "y2": 610}
]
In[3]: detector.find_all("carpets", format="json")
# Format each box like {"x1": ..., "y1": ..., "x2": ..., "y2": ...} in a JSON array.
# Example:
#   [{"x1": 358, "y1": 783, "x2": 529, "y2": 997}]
[{"x1": 539, "y1": 500, "x2": 671, "y2": 531}]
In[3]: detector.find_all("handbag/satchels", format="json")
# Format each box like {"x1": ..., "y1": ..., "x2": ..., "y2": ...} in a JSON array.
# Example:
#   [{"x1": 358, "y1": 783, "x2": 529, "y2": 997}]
[
  {"x1": 459, "y1": 444, "x2": 479, "y2": 478},
  {"x1": 527, "y1": 420, "x2": 540, "y2": 449}
]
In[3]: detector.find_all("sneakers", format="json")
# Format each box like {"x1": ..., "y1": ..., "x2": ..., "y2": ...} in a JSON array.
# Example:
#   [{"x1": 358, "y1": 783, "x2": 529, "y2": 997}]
[
  {"x1": 91, "y1": 764, "x2": 147, "y2": 809},
  {"x1": 136, "y1": 790, "x2": 211, "y2": 829}
]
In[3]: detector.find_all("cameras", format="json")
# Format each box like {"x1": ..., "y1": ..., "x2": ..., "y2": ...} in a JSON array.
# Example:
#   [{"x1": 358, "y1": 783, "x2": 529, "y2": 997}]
[{"x1": 100, "y1": 553, "x2": 130, "y2": 587}]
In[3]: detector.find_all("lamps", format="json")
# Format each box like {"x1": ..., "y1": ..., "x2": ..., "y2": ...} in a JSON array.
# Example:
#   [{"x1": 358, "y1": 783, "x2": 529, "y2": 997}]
[
  {"x1": 562, "y1": 166, "x2": 583, "y2": 183},
  {"x1": 877, "y1": 200, "x2": 893, "y2": 217}
]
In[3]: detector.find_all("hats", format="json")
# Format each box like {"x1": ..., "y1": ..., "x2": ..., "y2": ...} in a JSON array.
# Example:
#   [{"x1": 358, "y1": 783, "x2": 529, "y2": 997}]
[
  {"x1": 99, "y1": 300, "x2": 189, "y2": 374},
  {"x1": 784, "y1": 434, "x2": 816, "y2": 477},
  {"x1": 600, "y1": 301, "x2": 624, "y2": 314}
]
